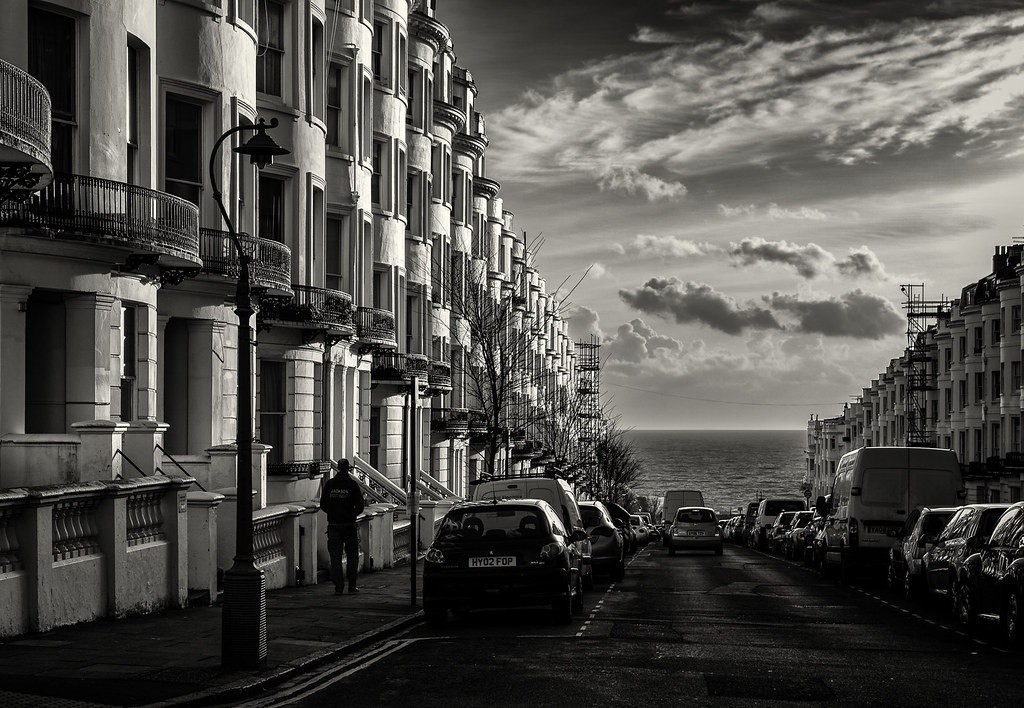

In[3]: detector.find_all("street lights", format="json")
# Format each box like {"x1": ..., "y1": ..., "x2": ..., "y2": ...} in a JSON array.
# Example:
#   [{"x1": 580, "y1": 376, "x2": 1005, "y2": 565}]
[{"x1": 209, "y1": 116, "x2": 294, "y2": 673}]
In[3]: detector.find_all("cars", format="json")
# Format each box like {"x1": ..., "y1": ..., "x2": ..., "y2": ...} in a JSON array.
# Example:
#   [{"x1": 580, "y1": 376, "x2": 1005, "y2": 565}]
[
  {"x1": 575, "y1": 500, "x2": 627, "y2": 581},
  {"x1": 721, "y1": 493, "x2": 831, "y2": 567},
  {"x1": 963, "y1": 500, "x2": 1024, "y2": 651},
  {"x1": 422, "y1": 497, "x2": 589, "y2": 626},
  {"x1": 665, "y1": 506, "x2": 725, "y2": 556},
  {"x1": 606, "y1": 501, "x2": 664, "y2": 552},
  {"x1": 917, "y1": 503, "x2": 1013, "y2": 615},
  {"x1": 890, "y1": 504, "x2": 960, "y2": 602}
]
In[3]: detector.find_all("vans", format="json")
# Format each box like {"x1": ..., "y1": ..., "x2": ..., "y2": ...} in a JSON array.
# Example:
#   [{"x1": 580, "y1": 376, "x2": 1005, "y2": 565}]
[
  {"x1": 663, "y1": 490, "x2": 706, "y2": 546},
  {"x1": 813, "y1": 445, "x2": 968, "y2": 586},
  {"x1": 471, "y1": 465, "x2": 601, "y2": 593}
]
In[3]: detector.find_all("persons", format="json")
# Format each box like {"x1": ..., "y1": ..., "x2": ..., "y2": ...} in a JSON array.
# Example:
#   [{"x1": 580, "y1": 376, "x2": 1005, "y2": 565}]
[{"x1": 319, "y1": 458, "x2": 365, "y2": 594}]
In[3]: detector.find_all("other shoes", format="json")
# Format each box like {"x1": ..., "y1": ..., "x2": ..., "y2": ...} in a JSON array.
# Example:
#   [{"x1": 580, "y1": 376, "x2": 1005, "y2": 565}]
[
  {"x1": 334, "y1": 589, "x2": 343, "y2": 595},
  {"x1": 349, "y1": 587, "x2": 359, "y2": 593}
]
